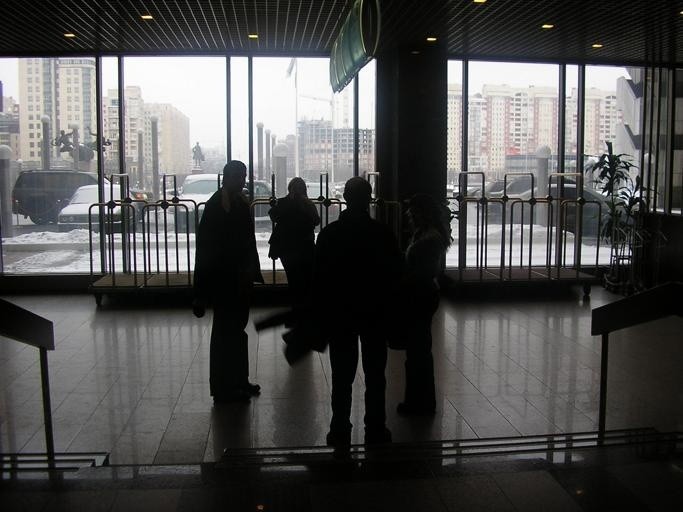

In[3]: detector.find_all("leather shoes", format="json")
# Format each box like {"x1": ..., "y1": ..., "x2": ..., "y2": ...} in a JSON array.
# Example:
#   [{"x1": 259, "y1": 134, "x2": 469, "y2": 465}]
[
  {"x1": 326, "y1": 427, "x2": 352, "y2": 447},
  {"x1": 397, "y1": 400, "x2": 437, "y2": 416},
  {"x1": 212, "y1": 383, "x2": 260, "y2": 404},
  {"x1": 365, "y1": 429, "x2": 393, "y2": 443}
]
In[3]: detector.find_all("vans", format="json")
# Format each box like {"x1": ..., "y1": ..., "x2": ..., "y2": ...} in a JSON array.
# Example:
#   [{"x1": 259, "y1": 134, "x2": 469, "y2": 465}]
[{"x1": 11, "y1": 170, "x2": 154, "y2": 225}]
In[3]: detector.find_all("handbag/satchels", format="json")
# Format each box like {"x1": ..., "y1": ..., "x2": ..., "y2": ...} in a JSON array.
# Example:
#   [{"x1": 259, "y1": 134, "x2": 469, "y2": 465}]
[{"x1": 384, "y1": 273, "x2": 418, "y2": 350}]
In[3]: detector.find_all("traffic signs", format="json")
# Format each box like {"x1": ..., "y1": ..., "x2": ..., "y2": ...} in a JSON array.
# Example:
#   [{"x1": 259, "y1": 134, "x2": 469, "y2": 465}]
[
  {"x1": 150, "y1": 115, "x2": 160, "y2": 211},
  {"x1": 70, "y1": 122, "x2": 80, "y2": 171},
  {"x1": 136, "y1": 129, "x2": 146, "y2": 189}
]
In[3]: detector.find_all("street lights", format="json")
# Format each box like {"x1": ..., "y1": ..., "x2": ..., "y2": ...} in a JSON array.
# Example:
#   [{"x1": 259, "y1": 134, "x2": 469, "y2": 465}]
[
  {"x1": 566, "y1": 158, "x2": 578, "y2": 181},
  {"x1": 0, "y1": 145, "x2": 13, "y2": 238},
  {"x1": 585, "y1": 158, "x2": 597, "y2": 193},
  {"x1": 532, "y1": 144, "x2": 552, "y2": 230},
  {"x1": 256, "y1": 121, "x2": 290, "y2": 204},
  {"x1": 39, "y1": 115, "x2": 54, "y2": 172}
]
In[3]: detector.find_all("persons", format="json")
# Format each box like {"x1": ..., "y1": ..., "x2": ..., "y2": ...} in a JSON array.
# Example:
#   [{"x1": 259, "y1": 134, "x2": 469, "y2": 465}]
[
  {"x1": 191, "y1": 159, "x2": 265, "y2": 402},
  {"x1": 312, "y1": 178, "x2": 405, "y2": 455},
  {"x1": 267, "y1": 177, "x2": 320, "y2": 286},
  {"x1": 396, "y1": 190, "x2": 453, "y2": 421}
]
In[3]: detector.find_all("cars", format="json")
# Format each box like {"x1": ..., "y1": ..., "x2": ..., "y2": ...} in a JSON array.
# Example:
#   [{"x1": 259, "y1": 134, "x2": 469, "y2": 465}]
[
  {"x1": 57, "y1": 184, "x2": 140, "y2": 233},
  {"x1": 305, "y1": 183, "x2": 338, "y2": 225},
  {"x1": 467, "y1": 175, "x2": 626, "y2": 230},
  {"x1": 176, "y1": 174, "x2": 224, "y2": 223},
  {"x1": 247, "y1": 180, "x2": 275, "y2": 217}
]
